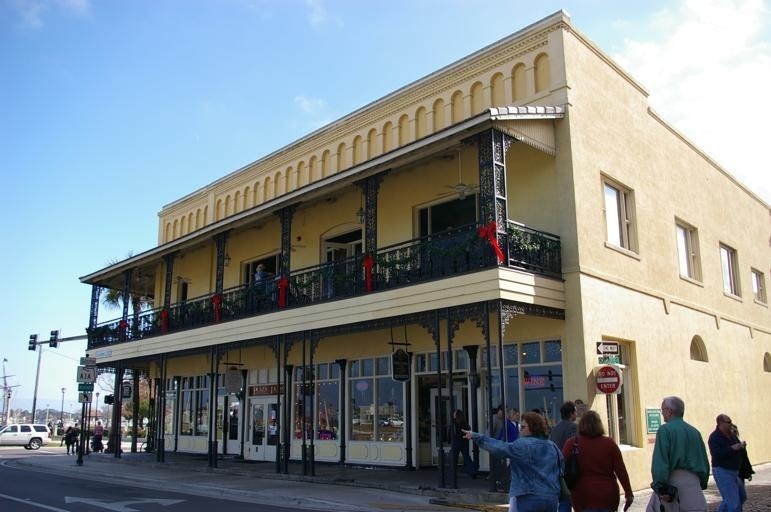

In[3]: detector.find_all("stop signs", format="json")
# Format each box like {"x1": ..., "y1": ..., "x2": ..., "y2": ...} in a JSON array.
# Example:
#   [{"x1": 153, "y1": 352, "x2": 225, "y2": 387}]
[{"x1": 595, "y1": 366, "x2": 621, "y2": 393}]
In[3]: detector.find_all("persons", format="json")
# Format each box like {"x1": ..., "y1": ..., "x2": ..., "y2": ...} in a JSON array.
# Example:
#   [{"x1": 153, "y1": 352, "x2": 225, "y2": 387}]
[
  {"x1": 646, "y1": 397, "x2": 710, "y2": 512},
  {"x1": 707, "y1": 414, "x2": 746, "y2": 512},
  {"x1": 72, "y1": 424, "x2": 79, "y2": 453},
  {"x1": 94, "y1": 422, "x2": 103, "y2": 442},
  {"x1": 65, "y1": 427, "x2": 73, "y2": 455},
  {"x1": 90, "y1": 439, "x2": 104, "y2": 453},
  {"x1": 448, "y1": 400, "x2": 635, "y2": 512},
  {"x1": 730, "y1": 424, "x2": 755, "y2": 482},
  {"x1": 254, "y1": 264, "x2": 274, "y2": 289}
]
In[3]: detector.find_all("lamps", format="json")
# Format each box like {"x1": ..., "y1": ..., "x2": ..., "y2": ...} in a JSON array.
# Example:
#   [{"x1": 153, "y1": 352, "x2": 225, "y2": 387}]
[
  {"x1": 224, "y1": 244, "x2": 231, "y2": 267},
  {"x1": 355, "y1": 192, "x2": 365, "y2": 224}
]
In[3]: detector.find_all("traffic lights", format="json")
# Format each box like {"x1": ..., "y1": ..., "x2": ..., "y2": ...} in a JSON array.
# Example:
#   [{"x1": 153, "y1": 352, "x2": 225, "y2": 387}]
[
  {"x1": 49, "y1": 330, "x2": 58, "y2": 348},
  {"x1": 29, "y1": 333, "x2": 37, "y2": 351}
]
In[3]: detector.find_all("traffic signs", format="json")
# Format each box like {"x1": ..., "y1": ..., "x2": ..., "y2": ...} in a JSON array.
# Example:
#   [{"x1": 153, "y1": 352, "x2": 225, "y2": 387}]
[
  {"x1": 80, "y1": 357, "x2": 96, "y2": 364},
  {"x1": 597, "y1": 341, "x2": 618, "y2": 354},
  {"x1": 79, "y1": 393, "x2": 92, "y2": 403},
  {"x1": 77, "y1": 367, "x2": 96, "y2": 382},
  {"x1": 79, "y1": 384, "x2": 94, "y2": 391},
  {"x1": 390, "y1": 348, "x2": 410, "y2": 383},
  {"x1": 597, "y1": 356, "x2": 620, "y2": 364}
]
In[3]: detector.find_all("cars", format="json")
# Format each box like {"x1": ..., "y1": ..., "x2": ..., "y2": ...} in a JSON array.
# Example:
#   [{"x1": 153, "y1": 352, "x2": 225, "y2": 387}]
[
  {"x1": 353, "y1": 415, "x2": 360, "y2": 426},
  {"x1": 378, "y1": 417, "x2": 404, "y2": 426},
  {"x1": 47, "y1": 415, "x2": 147, "y2": 428}
]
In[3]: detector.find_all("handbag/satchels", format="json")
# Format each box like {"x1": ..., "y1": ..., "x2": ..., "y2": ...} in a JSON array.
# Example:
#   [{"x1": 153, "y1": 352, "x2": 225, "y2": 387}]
[
  {"x1": 554, "y1": 445, "x2": 571, "y2": 498},
  {"x1": 565, "y1": 435, "x2": 580, "y2": 482}
]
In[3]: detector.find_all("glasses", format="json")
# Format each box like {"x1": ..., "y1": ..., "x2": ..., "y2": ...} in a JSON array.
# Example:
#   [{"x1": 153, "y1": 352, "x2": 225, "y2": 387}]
[
  {"x1": 520, "y1": 424, "x2": 528, "y2": 431},
  {"x1": 724, "y1": 421, "x2": 731, "y2": 424}
]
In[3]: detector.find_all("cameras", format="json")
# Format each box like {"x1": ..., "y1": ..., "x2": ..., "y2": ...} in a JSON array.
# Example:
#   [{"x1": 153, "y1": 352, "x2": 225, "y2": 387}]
[{"x1": 650, "y1": 480, "x2": 677, "y2": 503}]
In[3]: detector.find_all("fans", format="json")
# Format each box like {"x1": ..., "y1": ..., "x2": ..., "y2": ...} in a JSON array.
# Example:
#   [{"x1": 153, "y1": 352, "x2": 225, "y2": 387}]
[
  {"x1": 173, "y1": 255, "x2": 192, "y2": 284},
  {"x1": 435, "y1": 146, "x2": 480, "y2": 199}
]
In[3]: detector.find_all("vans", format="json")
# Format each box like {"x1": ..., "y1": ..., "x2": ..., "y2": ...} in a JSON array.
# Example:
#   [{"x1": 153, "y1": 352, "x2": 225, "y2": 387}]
[{"x1": 0, "y1": 422, "x2": 52, "y2": 450}]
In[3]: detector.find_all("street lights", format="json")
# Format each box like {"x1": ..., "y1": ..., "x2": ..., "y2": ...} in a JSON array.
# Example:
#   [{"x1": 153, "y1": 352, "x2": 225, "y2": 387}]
[
  {"x1": 46, "y1": 403, "x2": 50, "y2": 420},
  {"x1": 5, "y1": 387, "x2": 13, "y2": 424},
  {"x1": 61, "y1": 387, "x2": 67, "y2": 421},
  {"x1": 95, "y1": 392, "x2": 99, "y2": 426}
]
